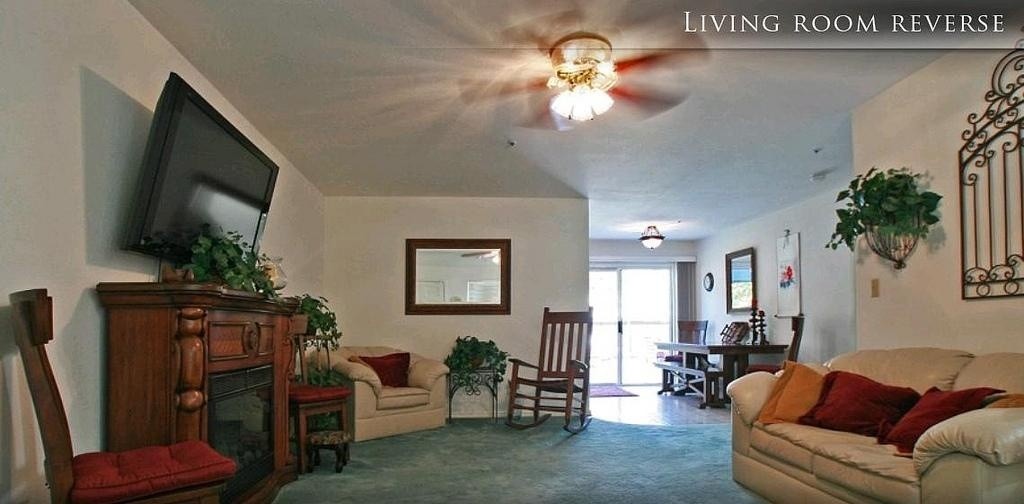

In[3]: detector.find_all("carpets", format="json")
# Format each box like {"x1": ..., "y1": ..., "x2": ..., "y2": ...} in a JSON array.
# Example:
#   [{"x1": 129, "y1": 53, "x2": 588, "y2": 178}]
[{"x1": 587, "y1": 382, "x2": 640, "y2": 398}]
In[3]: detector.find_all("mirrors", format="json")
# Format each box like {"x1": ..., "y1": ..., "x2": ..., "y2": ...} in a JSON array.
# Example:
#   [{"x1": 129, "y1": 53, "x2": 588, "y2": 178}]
[
  {"x1": 405, "y1": 238, "x2": 511, "y2": 315},
  {"x1": 725, "y1": 248, "x2": 757, "y2": 316}
]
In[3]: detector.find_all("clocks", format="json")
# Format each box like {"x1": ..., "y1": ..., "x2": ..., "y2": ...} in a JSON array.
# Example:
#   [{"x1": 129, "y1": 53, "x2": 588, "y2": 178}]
[{"x1": 702, "y1": 272, "x2": 713, "y2": 294}]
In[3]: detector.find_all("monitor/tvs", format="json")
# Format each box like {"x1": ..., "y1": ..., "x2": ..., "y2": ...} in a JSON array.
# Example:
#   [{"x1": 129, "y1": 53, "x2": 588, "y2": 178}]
[{"x1": 124, "y1": 72, "x2": 280, "y2": 272}]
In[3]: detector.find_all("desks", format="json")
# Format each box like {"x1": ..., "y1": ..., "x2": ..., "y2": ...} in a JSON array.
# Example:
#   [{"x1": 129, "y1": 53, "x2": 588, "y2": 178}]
[
  {"x1": 448, "y1": 364, "x2": 499, "y2": 424},
  {"x1": 649, "y1": 341, "x2": 787, "y2": 408}
]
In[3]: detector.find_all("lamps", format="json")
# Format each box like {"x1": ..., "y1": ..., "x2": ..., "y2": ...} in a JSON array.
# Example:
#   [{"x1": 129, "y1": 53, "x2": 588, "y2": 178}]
[
  {"x1": 545, "y1": 31, "x2": 616, "y2": 124},
  {"x1": 638, "y1": 225, "x2": 666, "y2": 253}
]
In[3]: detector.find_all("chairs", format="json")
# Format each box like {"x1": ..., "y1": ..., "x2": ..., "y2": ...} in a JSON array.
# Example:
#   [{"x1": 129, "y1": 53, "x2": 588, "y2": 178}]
[
  {"x1": 663, "y1": 319, "x2": 707, "y2": 390},
  {"x1": 505, "y1": 307, "x2": 593, "y2": 435},
  {"x1": 288, "y1": 315, "x2": 352, "y2": 475},
  {"x1": 8, "y1": 287, "x2": 237, "y2": 504},
  {"x1": 744, "y1": 313, "x2": 803, "y2": 375}
]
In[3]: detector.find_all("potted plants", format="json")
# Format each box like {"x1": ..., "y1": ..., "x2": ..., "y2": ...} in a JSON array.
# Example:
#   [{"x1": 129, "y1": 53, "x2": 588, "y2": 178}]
[
  {"x1": 444, "y1": 333, "x2": 508, "y2": 395},
  {"x1": 820, "y1": 162, "x2": 940, "y2": 271},
  {"x1": 285, "y1": 291, "x2": 343, "y2": 352}
]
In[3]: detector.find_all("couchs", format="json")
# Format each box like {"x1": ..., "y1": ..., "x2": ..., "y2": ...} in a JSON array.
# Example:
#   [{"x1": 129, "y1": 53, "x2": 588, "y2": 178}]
[
  {"x1": 726, "y1": 346, "x2": 1024, "y2": 504},
  {"x1": 312, "y1": 343, "x2": 452, "y2": 444}
]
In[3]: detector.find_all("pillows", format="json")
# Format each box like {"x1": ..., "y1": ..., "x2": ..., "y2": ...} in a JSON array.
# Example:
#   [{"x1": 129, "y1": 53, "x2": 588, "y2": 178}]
[
  {"x1": 757, "y1": 358, "x2": 1023, "y2": 460},
  {"x1": 349, "y1": 352, "x2": 409, "y2": 387}
]
